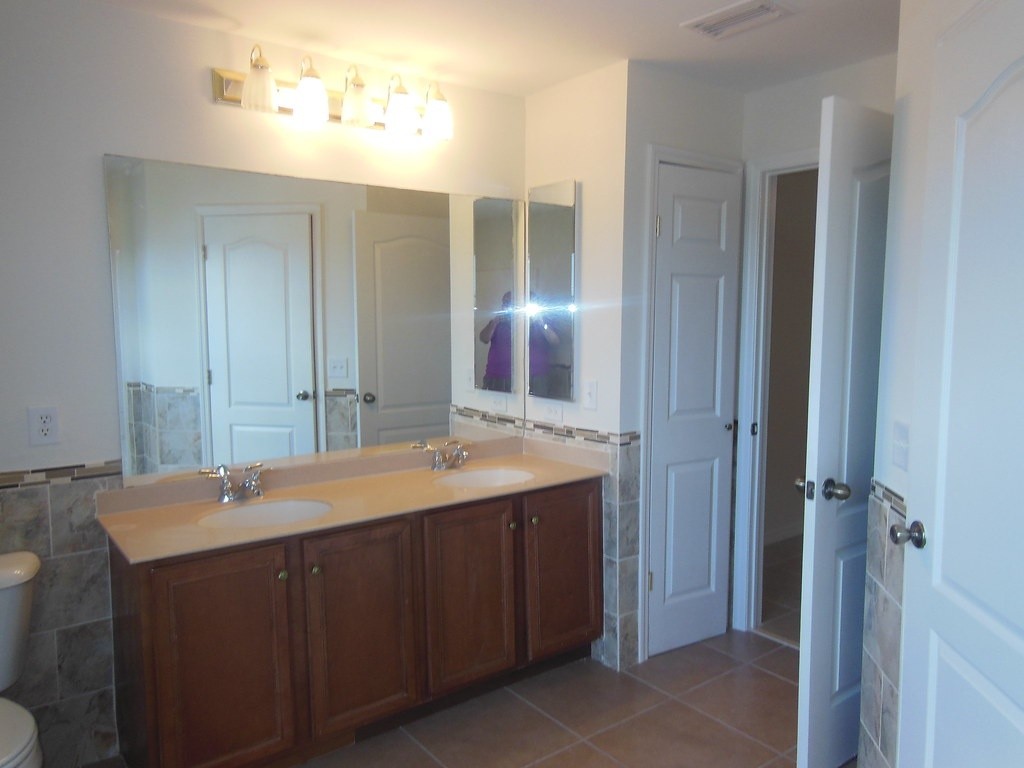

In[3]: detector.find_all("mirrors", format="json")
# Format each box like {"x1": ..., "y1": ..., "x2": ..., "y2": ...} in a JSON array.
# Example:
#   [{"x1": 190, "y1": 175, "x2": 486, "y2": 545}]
[
  {"x1": 102, "y1": 152, "x2": 450, "y2": 476},
  {"x1": 528, "y1": 178, "x2": 578, "y2": 404},
  {"x1": 473, "y1": 197, "x2": 515, "y2": 395}
]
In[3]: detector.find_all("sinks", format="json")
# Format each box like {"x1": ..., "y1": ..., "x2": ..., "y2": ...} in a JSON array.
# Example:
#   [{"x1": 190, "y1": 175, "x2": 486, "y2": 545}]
[
  {"x1": 156, "y1": 466, "x2": 247, "y2": 483},
  {"x1": 360, "y1": 444, "x2": 420, "y2": 454},
  {"x1": 431, "y1": 467, "x2": 537, "y2": 488},
  {"x1": 195, "y1": 499, "x2": 335, "y2": 530}
]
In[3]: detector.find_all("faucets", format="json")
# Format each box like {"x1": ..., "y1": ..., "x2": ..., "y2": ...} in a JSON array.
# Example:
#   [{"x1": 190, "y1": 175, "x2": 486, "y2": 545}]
[
  {"x1": 415, "y1": 438, "x2": 438, "y2": 450},
  {"x1": 445, "y1": 449, "x2": 471, "y2": 471},
  {"x1": 215, "y1": 464, "x2": 231, "y2": 474},
  {"x1": 232, "y1": 476, "x2": 264, "y2": 503}
]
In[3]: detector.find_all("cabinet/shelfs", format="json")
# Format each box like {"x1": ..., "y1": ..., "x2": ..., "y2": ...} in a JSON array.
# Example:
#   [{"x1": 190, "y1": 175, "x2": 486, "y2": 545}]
[
  {"x1": 415, "y1": 475, "x2": 603, "y2": 717},
  {"x1": 107, "y1": 514, "x2": 422, "y2": 768}
]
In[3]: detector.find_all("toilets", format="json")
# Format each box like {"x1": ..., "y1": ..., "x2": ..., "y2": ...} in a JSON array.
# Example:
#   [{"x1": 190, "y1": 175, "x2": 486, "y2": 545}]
[{"x1": 0, "y1": 552, "x2": 44, "y2": 768}]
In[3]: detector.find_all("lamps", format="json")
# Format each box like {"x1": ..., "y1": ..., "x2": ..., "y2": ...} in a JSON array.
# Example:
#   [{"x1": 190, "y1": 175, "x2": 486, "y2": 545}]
[{"x1": 211, "y1": 44, "x2": 455, "y2": 144}]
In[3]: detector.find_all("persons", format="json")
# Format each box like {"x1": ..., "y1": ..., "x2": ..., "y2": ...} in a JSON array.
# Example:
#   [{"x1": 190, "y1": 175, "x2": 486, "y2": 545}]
[{"x1": 479, "y1": 292, "x2": 562, "y2": 397}]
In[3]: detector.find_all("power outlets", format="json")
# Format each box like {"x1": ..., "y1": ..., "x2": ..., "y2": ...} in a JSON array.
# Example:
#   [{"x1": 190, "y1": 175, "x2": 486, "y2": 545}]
[{"x1": 27, "y1": 402, "x2": 63, "y2": 447}]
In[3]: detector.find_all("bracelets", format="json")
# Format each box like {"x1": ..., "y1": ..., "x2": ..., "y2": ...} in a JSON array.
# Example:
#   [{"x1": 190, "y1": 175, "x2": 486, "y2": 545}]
[{"x1": 541, "y1": 324, "x2": 547, "y2": 330}]
